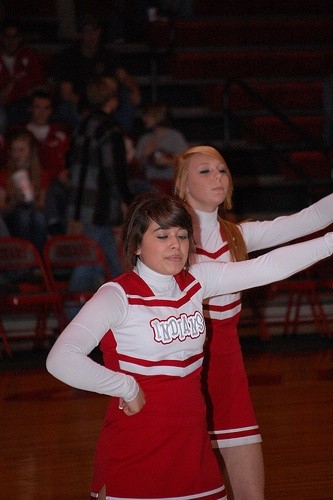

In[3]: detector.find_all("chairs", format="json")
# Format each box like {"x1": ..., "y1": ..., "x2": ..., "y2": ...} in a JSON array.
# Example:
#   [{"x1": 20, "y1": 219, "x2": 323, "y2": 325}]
[
  {"x1": 262, "y1": 257, "x2": 333, "y2": 339},
  {"x1": 0, "y1": 235, "x2": 113, "y2": 364}
]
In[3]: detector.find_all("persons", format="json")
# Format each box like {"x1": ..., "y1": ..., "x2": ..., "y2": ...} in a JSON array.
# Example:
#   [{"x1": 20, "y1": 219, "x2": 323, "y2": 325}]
[
  {"x1": 45, "y1": 189, "x2": 333, "y2": 500},
  {"x1": 0, "y1": 12, "x2": 189, "y2": 346},
  {"x1": 100, "y1": 145, "x2": 333, "y2": 500}
]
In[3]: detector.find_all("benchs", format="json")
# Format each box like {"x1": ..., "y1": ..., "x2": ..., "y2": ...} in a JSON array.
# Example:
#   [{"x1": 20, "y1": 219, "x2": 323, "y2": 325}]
[{"x1": 153, "y1": 0, "x2": 333, "y2": 203}]
